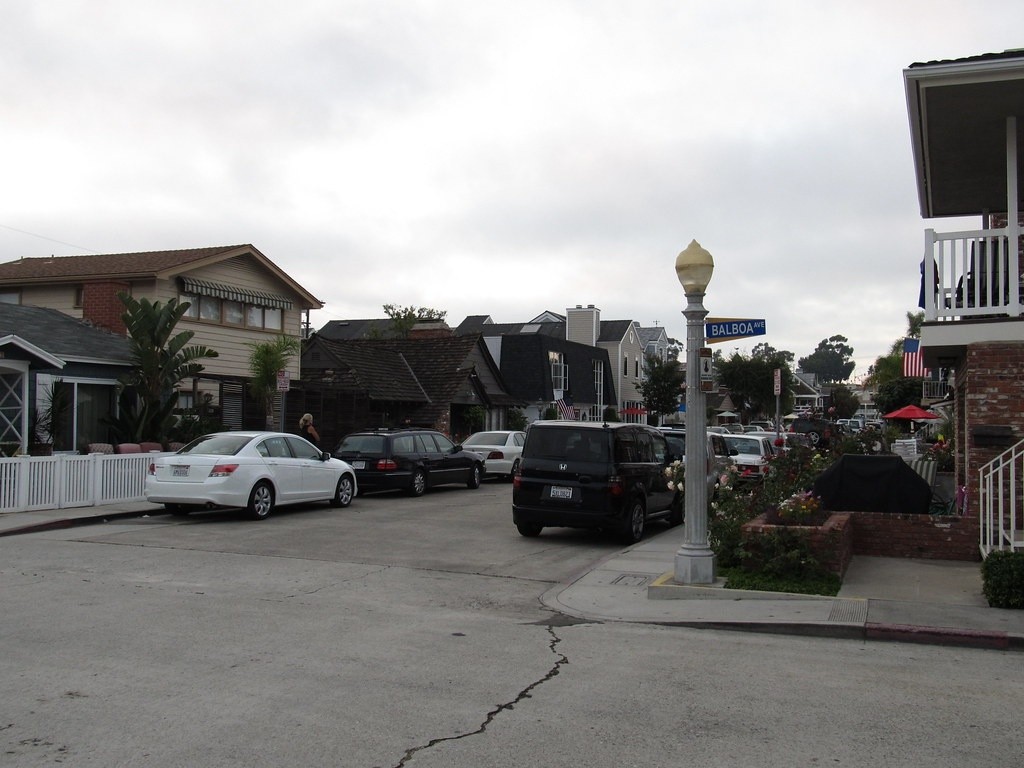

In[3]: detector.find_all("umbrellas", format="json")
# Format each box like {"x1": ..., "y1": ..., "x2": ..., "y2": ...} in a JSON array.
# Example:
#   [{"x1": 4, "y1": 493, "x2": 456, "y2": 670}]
[
  {"x1": 617, "y1": 408, "x2": 648, "y2": 423},
  {"x1": 716, "y1": 410, "x2": 738, "y2": 423},
  {"x1": 881, "y1": 404, "x2": 942, "y2": 440},
  {"x1": 642, "y1": 405, "x2": 659, "y2": 414}
]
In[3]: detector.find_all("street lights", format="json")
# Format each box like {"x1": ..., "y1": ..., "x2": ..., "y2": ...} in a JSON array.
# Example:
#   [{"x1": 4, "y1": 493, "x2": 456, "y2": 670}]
[{"x1": 674, "y1": 238, "x2": 718, "y2": 585}]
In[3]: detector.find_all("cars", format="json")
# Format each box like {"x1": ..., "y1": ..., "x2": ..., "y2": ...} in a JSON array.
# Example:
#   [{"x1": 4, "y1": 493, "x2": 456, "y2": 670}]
[
  {"x1": 459, "y1": 429, "x2": 527, "y2": 481},
  {"x1": 655, "y1": 404, "x2": 887, "y2": 502},
  {"x1": 143, "y1": 431, "x2": 359, "y2": 520}
]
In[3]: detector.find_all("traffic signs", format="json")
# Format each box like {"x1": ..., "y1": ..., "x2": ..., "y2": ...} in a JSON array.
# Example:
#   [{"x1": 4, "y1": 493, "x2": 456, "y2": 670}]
[{"x1": 706, "y1": 316, "x2": 767, "y2": 346}]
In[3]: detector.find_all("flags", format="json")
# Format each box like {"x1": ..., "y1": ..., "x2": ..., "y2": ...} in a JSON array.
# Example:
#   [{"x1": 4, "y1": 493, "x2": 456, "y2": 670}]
[
  {"x1": 556, "y1": 396, "x2": 576, "y2": 420},
  {"x1": 903, "y1": 337, "x2": 932, "y2": 378}
]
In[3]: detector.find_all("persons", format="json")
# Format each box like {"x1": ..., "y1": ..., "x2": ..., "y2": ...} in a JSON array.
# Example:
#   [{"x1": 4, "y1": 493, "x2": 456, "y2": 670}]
[{"x1": 299, "y1": 413, "x2": 320, "y2": 446}]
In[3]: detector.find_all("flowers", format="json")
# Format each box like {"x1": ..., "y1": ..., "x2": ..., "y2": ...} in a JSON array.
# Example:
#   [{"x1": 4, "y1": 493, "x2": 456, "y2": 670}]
[
  {"x1": 710, "y1": 439, "x2": 830, "y2": 518},
  {"x1": 664, "y1": 460, "x2": 685, "y2": 492}
]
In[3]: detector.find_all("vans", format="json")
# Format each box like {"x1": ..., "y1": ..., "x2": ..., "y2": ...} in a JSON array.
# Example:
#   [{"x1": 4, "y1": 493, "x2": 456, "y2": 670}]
[
  {"x1": 331, "y1": 429, "x2": 487, "y2": 498},
  {"x1": 509, "y1": 419, "x2": 688, "y2": 544}
]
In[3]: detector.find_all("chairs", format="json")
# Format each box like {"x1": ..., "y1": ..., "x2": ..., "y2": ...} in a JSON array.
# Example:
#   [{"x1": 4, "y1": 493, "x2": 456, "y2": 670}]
[
  {"x1": 946, "y1": 240, "x2": 1008, "y2": 308},
  {"x1": 910, "y1": 459, "x2": 938, "y2": 505},
  {"x1": 891, "y1": 442, "x2": 916, "y2": 467}
]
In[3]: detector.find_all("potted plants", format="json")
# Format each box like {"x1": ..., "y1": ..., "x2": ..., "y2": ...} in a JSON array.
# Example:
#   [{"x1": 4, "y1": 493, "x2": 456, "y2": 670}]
[{"x1": 28, "y1": 377, "x2": 69, "y2": 456}]
